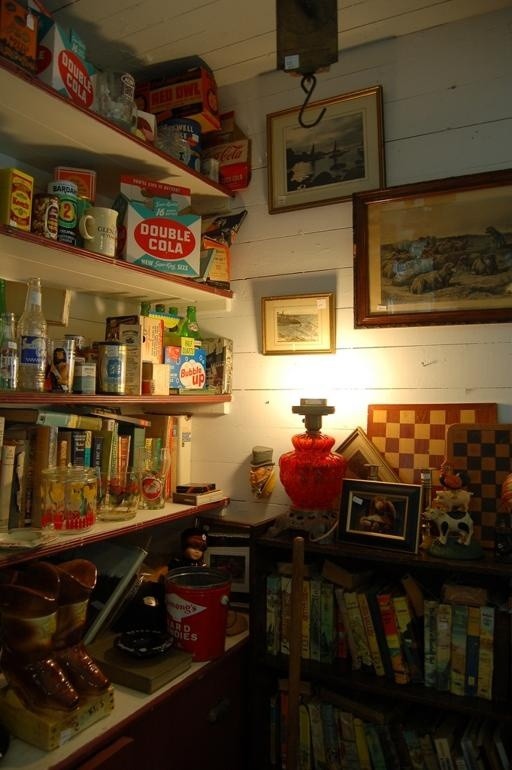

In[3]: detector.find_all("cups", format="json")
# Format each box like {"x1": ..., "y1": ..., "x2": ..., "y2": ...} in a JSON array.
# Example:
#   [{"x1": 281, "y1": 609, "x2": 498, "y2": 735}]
[
  {"x1": 140, "y1": 447, "x2": 170, "y2": 511},
  {"x1": 78, "y1": 206, "x2": 119, "y2": 258},
  {"x1": 100, "y1": 465, "x2": 142, "y2": 521}
]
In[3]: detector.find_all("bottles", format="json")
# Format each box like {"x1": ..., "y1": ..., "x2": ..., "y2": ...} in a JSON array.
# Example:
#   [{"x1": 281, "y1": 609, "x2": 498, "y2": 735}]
[
  {"x1": 0, "y1": 313, "x2": 18, "y2": 391},
  {"x1": 167, "y1": 307, "x2": 182, "y2": 336},
  {"x1": 52, "y1": 339, "x2": 75, "y2": 393},
  {"x1": 49, "y1": 181, "x2": 77, "y2": 244},
  {"x1": 98, "y1": 343, "x2": 127, "y2": 395},
  {"x1": 0, "y1": 279, "x2": 8, "y2": 341},
  {"x1": 181, "y1": 306, "x2": 201, "y2": 340},
  {"x1": 17, "y1": 278, "x2": 47, "y2": 392},
  {"x1": 31, "y1": 192, "x2": 58, "y2": 242},
  {"x1": 0, "y1": 168, "x2": 33, "y2": 230}
]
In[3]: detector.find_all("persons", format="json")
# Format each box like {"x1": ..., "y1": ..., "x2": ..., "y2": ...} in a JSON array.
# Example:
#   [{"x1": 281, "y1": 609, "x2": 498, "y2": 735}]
[
  {"x1": 176, "y1": 534, "x2": 208, "y2": 567},
  {"x1": 46, "y1": 348, "x2": 70, "y2": 393}
]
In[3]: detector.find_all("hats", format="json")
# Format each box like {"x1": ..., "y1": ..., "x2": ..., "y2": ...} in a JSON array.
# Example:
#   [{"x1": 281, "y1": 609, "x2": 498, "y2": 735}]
[{"x1": 180, "y1": 528, "x2": 208, "y2": 548}]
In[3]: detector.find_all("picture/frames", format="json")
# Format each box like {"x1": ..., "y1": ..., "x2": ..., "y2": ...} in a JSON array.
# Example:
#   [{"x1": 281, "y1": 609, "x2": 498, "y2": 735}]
[
  {"x1": 265, "y1": 84, "x2": 384, "y2": 213},
  {"x1": 260, "y1": 291, "x2": 336, "y2": 356},
  {"x1": 335, "y1": 479, "x2": 421, "y2": 556},
  {"x1": 200, "y1": 536, "x2": 252, "y2": 600},
  {"x1": 352, "y1": 168, "x2": 512, "y2": 330},
  {"x1": 336, "y1": 426, "x2": 401, "y2": 482}
]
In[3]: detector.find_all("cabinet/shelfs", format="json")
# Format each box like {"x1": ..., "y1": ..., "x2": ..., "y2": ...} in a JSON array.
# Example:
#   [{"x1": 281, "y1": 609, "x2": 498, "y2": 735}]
[
  {"x1": 0, "y1": 59, "x2": 251, "y2": 769},
  {"x1": 196, "y1": 503, "x2": 288, "y2": 651},
  {"x1": 253, "y1": 529, "x2": 511, "y2": 769}
]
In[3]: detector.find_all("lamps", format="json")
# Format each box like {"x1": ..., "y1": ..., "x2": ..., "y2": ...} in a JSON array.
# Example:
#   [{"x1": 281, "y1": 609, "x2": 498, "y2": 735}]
[{"x1": 268, "y1": 398, "x2": 346, "y2": 546}]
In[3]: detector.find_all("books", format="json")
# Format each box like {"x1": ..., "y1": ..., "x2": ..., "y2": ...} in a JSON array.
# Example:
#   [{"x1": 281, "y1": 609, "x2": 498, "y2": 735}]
[
  {"x1": 0, "y1": 406, "x2": 193, "y2": 534},
  {"x1": 299, "y1": 701, "x2": 512, "y2": 769},
  {"x1": 265, "y1": 559, "x2": 494, "y2": 700}
]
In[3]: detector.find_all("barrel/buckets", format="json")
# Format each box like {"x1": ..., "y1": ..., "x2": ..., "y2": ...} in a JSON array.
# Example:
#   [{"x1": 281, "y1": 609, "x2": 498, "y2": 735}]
[{"x1": 164, "y1": 566, "x2": 231, "y2": 662}]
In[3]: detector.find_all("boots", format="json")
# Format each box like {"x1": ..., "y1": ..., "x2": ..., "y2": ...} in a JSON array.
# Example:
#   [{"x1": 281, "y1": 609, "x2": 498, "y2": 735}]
[
  {"x1": 0, "y1": 561, "x2": 79, "y2": 718},
  {"x1": 53, "y1": 558, "x2": 110, "y2": 698}
]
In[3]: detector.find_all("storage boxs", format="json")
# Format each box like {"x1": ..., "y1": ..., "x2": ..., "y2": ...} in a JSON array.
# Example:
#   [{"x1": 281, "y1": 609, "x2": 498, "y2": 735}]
[
  {"x1": 137, "y1": 66, "x2": 222, "y2": 133},
  {"x1": 0, "y1": 1, "x2": 40, "y2": 75},
  {"x1": 199, "y1": 235, "x2": 231, "y2": 290},
  {"x1": 36, "y1": 24, "x2": 99, "y2": 115},
  {"x1": 111, "y1": 174, "x2": 202, "y2": 280}
]
